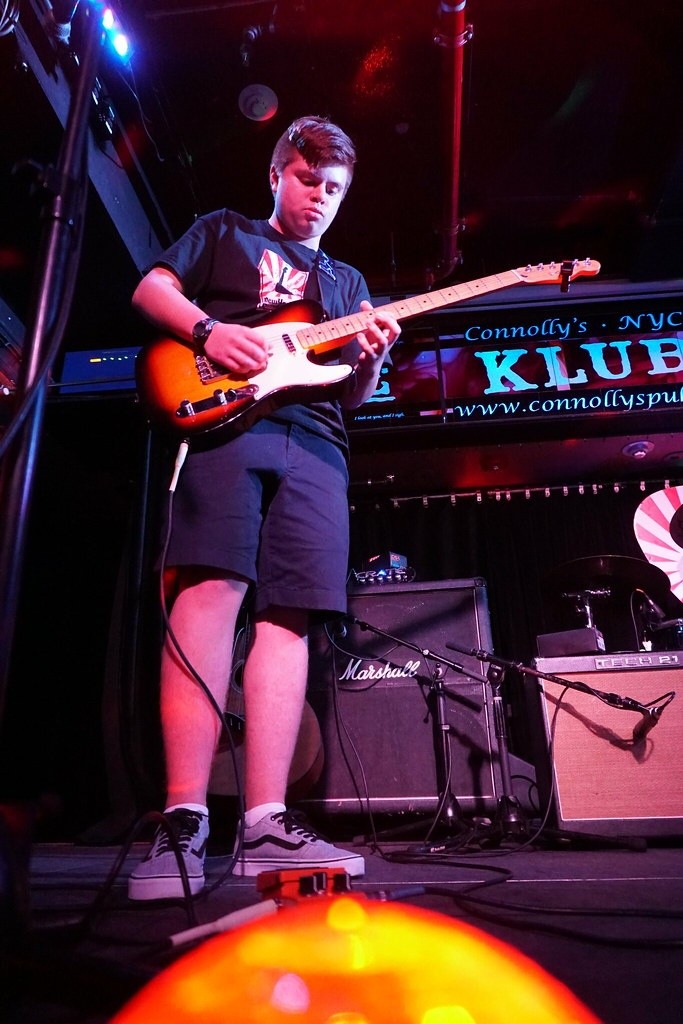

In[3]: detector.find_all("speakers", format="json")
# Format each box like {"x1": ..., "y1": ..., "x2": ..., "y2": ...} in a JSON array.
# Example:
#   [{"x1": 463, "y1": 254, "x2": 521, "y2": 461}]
[
  {"x1": 288, "y1": 576, "x2": 507, "y2": 815},
  {"x1": 527, "y1": 650, "x2": 681, "y2": 845}
]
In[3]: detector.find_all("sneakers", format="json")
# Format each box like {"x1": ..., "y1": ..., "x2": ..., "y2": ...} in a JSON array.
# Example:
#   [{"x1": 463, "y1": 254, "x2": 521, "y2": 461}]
[
  {"x1": 232, "y1": 807, "x2": 365, "y2": 876},
  {"x1": 128, "y1": 809, "x2": 210, "y2": 900}
]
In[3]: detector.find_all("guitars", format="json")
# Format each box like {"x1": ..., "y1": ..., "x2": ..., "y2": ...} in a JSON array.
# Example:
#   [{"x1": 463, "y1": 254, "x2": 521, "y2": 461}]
[
  {"x1": 208, "y1": 598, "x2": 327, "y2": 799},
  {"x1": 134, "y1": 258, "x2": 604, "y2": 453}
]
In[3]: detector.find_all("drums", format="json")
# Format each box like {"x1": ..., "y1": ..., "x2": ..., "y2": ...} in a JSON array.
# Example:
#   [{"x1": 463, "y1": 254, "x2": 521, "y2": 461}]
[{"x1": 652, "y1": 619, "x2": 683, "y2": 651}]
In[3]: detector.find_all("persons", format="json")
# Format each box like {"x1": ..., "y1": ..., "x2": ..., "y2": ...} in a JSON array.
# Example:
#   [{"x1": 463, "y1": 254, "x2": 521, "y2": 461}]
[{"x1": 126, "y1": 116, "x2": 401, "y2": 901}]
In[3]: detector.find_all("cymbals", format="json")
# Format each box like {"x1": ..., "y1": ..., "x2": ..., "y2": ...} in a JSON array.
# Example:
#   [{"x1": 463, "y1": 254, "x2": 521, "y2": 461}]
[{"x1": 551, "y1": 554, "x2": 673, "y2": 609}]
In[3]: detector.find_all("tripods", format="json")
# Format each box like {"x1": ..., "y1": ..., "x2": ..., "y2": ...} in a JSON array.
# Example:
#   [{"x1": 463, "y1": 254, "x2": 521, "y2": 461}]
[{"x1": 346, "y1": 613, "x2": 654, "y2": 858}]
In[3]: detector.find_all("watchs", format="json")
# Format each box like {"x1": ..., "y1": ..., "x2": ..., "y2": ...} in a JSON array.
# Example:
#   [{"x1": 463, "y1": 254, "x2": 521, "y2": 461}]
[{"x1": 192, "y1": 318, "x2": 221, "y2": 357}]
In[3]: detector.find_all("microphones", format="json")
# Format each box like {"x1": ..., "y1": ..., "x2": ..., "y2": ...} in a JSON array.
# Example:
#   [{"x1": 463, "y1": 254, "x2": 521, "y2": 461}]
[
  {"x1": 328, "y1": 619, "x2": 347, "y2": 638},
  {"x1": 644, "y1": 595, "x2": 665, "y2": 620},
  {"x1": 632, "y1": 705, "x2": 662, "y2": 741}
]
